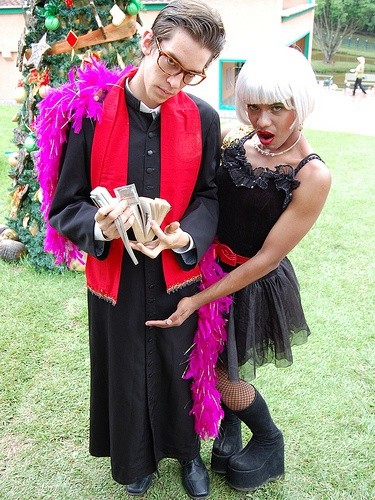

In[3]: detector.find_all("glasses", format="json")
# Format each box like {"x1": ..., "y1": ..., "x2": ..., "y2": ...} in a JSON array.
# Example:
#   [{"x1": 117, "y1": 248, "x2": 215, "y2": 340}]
[{"x1": 155, "y1": 33, "x2": 206, "y2": 86}]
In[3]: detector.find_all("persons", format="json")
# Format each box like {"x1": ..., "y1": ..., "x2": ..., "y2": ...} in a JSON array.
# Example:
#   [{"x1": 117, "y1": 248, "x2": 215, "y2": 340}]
[
  {"x1": 350, "y1": 56, "x2": 368, "y2": 97},
  {"x1": 49, "y1": 0, "x2": 227, "y2": 500},
  {"x1": 144, "y1": 41, "x2": 333, "y2": 491}
]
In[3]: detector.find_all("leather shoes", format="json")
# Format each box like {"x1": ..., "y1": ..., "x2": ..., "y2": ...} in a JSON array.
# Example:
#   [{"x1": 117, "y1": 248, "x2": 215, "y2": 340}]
[
  {"x1": 182, "y1": 454, "x2": 210, "y2": 498},
  {"x1": 127, "y1": 474, "x2": 152, "y2": 496}
]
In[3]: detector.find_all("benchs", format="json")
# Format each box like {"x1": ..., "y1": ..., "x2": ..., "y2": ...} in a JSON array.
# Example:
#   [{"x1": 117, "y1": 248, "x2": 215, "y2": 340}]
[{"x1": 343, "y1": 73, "x2": 375, "y2": 96}]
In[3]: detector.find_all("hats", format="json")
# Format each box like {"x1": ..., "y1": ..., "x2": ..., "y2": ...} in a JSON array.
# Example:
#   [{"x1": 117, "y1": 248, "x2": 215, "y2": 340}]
[{"x1": 357, "y1": 57, "x2": 365, "y2": 64}]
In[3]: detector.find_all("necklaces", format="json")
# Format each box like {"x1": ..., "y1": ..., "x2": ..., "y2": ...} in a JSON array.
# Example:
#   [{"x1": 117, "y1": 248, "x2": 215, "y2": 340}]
[{"x1": 252, "y1": 127, "x2": 302, "y2": 158}]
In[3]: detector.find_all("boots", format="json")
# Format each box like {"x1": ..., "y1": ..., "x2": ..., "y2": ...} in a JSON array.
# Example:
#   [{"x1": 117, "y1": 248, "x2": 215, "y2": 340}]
[
  {"x1": 226, "y1": 384, "x2": 284, "y2": 490},
  {"x1": 211, "y1": 402, "x2": 242, "y2": 474}
]
